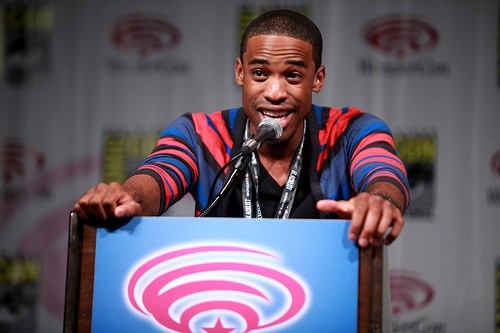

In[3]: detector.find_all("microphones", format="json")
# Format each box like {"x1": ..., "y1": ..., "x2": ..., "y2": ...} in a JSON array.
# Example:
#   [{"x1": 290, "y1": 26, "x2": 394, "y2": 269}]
[{"x1": 240, "y1": 118, "x2": 283, "y2": 156}]
